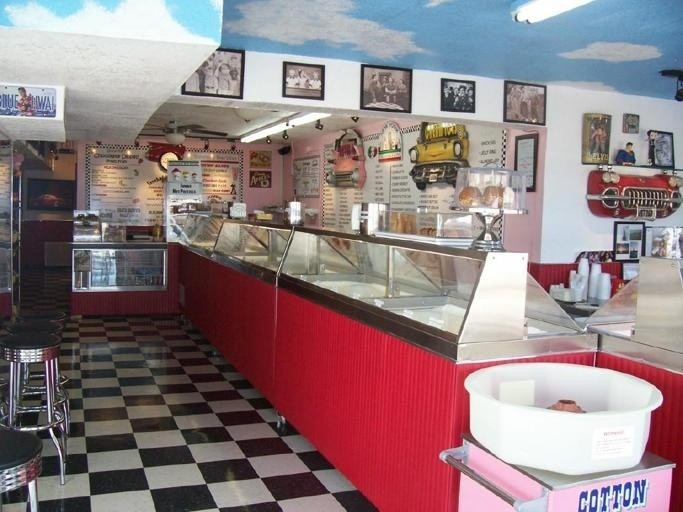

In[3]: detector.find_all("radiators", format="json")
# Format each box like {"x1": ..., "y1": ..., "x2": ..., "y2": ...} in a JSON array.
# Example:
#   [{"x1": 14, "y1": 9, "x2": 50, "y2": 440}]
[{"x1": 42, "y1": 242, "x2": 72, "y2": 268}]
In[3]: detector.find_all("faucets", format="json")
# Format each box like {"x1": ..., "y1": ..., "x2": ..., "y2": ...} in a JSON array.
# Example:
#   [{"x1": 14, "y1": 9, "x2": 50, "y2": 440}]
[{"x1": 126, "y1": 232, "x2": 134, "y2": 240}]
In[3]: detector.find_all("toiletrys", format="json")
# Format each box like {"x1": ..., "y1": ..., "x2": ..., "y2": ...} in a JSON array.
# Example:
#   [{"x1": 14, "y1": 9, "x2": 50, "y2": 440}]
[{"x1": 152, "y1": 215, "x2": 164, "y2": 242}]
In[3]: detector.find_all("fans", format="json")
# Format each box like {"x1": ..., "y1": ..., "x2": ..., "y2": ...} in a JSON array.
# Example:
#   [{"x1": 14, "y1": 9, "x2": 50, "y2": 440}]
[{"x1": 139, "y1": 120, "x2": 231, "y2": 145}]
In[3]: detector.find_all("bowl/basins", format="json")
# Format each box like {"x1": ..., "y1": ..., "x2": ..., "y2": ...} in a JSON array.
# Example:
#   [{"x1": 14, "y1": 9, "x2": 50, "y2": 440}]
[{"x1": 464, "y1": 362, "x2": 665, "y2": 477}]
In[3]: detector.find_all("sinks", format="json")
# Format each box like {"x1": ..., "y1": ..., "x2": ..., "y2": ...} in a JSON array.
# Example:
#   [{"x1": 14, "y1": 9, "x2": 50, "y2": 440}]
[{"x1": 127, "y1": 235, "x2": 153, "y2": 242}]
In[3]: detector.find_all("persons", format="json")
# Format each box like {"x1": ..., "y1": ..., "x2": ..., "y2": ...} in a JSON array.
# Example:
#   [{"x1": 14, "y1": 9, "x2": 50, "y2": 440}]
[
  {"x1": 615, "y1": 141, "x2": 636, "y2": 166},
  {"x1": 510, "y1": 85, "x2": 539, "y2": 123},
  {"x1": 443, "y1": 84, "x2": 473, "y2": 111},
  {"x1": 286, "y1": 68, "x2": 322, "y2": 90},
  {"x1": 17, "y1": 87, "x2": 34, "y2": 116},
  {"x1": 184, "y1": 53, "x2": 239, "y2": 95},
  {"x1": 369, "y1": 73, "x2": 406, "y2": 103}
]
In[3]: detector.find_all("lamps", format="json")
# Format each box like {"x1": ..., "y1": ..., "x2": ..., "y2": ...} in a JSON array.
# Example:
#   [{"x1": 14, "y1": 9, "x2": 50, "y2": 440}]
[
  {"x1": 239, "y1": 111, "x2": 360, "y2": 144},
  {"x1": 165, "y1": 133, "x2": 186, "y2": 145},
  {"x1": 513, "y1": 0, "x2": 592, "y2": 25}
]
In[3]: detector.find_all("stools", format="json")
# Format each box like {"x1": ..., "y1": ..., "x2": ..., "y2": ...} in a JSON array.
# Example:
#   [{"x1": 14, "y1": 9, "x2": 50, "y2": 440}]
[
  {"x1": 0, "y1": 307, "x2": 70, "y2": 485},
  {"x1": 1, "y1": 427, "x2": 46, "y2": 511}
]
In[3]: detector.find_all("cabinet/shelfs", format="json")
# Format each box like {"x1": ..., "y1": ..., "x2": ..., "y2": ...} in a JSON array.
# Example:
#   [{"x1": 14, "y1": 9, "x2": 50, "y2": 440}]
[
  {"x1": 590, "y1": 254, "x2": 683, "y2": 511},
  {"x1": 278, "y1": 220, "x2": 599, "y2": 510},
  {"x1": 69, "y1": 242, "x2": 179, "y2": 315},
  {"x1": 0, "y1": 146, "x2": 22, "y2": 316},
  {"x1": 433, "y1": 433, "x2": 674, "y2": 511},
  {"x1": 184, "y1": 213, "x2": 317, "y2": 406}
]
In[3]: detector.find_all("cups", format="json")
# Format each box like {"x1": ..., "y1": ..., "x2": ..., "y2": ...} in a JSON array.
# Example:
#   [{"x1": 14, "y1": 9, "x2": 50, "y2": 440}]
[{"x1": 569, "y1": 256, "x2": 613, "y2": 300}]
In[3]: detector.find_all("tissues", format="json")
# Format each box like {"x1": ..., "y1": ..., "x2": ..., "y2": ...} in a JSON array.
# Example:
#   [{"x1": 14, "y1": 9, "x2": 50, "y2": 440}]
[{"x1": 253, "y1": 209, "x2": 273, "y2": 220}]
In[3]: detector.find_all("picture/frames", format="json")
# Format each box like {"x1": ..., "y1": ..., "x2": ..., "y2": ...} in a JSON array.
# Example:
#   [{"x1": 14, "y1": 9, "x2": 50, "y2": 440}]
[
  {"x1": 612, "y1": 220, "x2": 647, "y2": 263},
  {"x1": 358, "y1": 64, "x2": 413, "y2": 114},
  {"x1": 181, "y1": 45, "x2": 247, "y2": 101},
  {"x1": 440, "y1": 76, "x2": 476, "y2": 113},
  {"x1": 501, "y1": 79, "x2": 547, "y2": 127},
  {"x1": 511, "y1": 133, "x2": 539, "y2": 193},
  {"x1": 650, "y1": 129, "x2": 676, "y2": 168},
  {"x1": 26, "y1": 177, "x2": 76, "y2": 212},
  {"x1": 282, "y1": 59, "x2": 325, "y2": 101}
]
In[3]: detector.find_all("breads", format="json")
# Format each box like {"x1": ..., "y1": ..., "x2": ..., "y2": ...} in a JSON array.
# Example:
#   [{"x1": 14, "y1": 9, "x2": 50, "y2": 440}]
[
  {"x1": 483, "y1": 186, "x2": 504, "y2": 208},
  {"x1": 460, "y1": 186, "x2": 482, "y2": 206}
]
